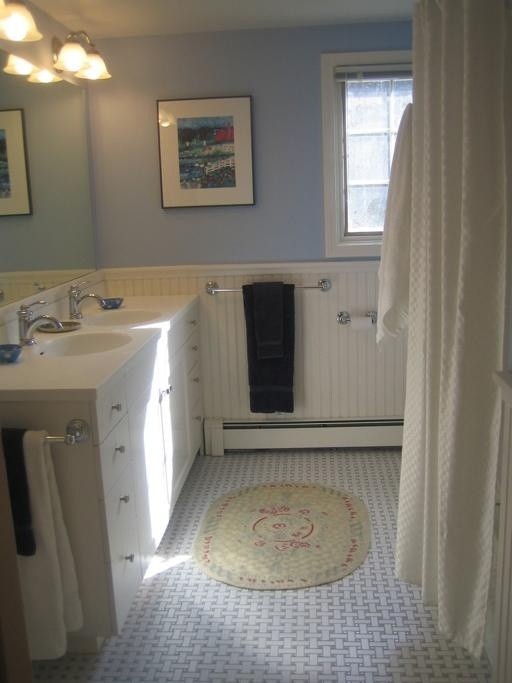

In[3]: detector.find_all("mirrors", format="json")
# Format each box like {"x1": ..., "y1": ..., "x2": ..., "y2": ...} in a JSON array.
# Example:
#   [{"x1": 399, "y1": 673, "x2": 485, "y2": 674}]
[{"x1": 0, "y1": 50, "x2": 99, "y2": 308}]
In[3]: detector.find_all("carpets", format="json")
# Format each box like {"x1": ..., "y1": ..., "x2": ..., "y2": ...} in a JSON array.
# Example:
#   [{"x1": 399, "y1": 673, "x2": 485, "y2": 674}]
[{"x1": 193, "y1": 481, "x2": 372, "y2": 590}]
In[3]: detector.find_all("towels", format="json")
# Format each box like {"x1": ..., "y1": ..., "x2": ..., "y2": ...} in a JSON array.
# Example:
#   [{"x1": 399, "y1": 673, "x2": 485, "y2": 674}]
[
  {"x1": 253, "y1": 280, "x2": 285, "y2": 360},
  {"x1": 1, "y1": 426, "x2": 37, "y2": 557},
  {"x1": 243, "y1": 285, "x2": 296, "y2": 414},
  {"x1": 16, "y1": 428, "x2": 82, "y2": 662}
]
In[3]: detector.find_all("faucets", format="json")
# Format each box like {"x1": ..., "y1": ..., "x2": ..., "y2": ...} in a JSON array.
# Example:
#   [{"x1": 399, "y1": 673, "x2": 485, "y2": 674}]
[
  {"x1": 17, "y1": 301, "x2": 64, "y2": 349},
  {"x1": 69, "y1": 281, "x2": 105, "y2": 319}
]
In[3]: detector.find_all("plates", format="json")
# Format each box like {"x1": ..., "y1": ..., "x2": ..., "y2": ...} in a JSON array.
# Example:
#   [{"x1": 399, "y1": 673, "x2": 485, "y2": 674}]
[{"x1": 38, "y1": 321, "x2": 81, "y2": 333}]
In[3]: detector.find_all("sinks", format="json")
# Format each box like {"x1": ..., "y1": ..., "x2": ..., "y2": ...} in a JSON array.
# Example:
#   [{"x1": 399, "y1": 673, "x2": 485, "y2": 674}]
[
  {"x1": 35, "y1": 333, "x2": 132, "y2": 358},
  {"x1": 82, "y1": 309, "x2": 162, "y2": 325}
]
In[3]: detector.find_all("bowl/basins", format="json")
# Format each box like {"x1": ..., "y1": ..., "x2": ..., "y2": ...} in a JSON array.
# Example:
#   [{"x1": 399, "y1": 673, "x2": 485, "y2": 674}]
[
  {"x1": 99, "y1": 298, "x2": 123, "y2": 309},
  {"x1": 0, "y1": 343, "x2": 24, "y2": 364}
]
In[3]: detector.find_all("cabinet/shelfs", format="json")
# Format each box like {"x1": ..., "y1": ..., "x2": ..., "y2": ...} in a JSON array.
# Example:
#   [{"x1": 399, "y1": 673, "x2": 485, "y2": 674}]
[{"x1": 0, "y1": 300, "x2": 203, "y2": 637}]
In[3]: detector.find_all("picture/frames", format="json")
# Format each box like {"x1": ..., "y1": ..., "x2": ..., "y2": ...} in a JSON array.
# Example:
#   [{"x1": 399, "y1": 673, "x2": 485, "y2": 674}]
[
  {"x1": 0, "y1": 110, "x2": 33, "y2": 216},
  {"x1": 157, "y1": 95, "x2": 255, "y2": 208}
]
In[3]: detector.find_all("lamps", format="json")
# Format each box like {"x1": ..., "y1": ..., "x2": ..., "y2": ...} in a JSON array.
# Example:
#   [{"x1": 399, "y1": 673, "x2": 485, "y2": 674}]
[
  {"x1": 2, "y1": 53, "x2": 60, "y2": 85},
  {"x1": 0, "y1": 2, "x2": 41, "y2": 42},
  {"x1": 54, "y1": 31, "x2": 112, "y2": 80}
]
